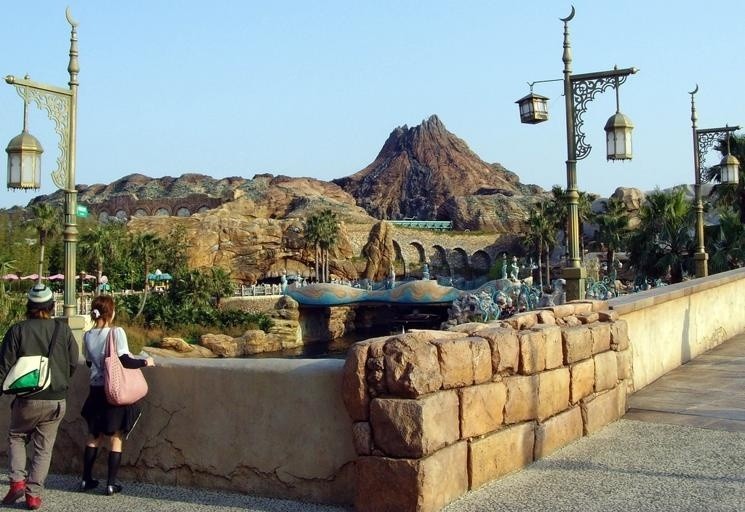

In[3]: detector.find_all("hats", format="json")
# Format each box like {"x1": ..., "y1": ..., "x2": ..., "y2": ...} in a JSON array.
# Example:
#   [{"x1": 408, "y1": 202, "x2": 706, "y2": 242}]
[{"x1": 26, "y1": 282, "x2": 54, "y2": 309}]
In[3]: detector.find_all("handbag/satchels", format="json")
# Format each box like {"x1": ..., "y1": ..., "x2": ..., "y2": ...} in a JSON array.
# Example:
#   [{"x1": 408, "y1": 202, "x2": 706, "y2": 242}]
[
  {"x1": 2, "y1": 318, "x2": 61, "y2": 399},
  {"x1": 105, "y1": 326, "x2": 150, "y2": 406}
]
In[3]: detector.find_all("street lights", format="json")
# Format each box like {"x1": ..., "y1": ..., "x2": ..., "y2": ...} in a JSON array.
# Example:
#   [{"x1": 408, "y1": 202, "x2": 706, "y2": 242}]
[
  {"x1": 515, "y1": 3, "x2": 640, "y2": 301},
  {"x1": 688, "y1": 83, "x2": 740, "y2": 278},
  {"x1": 1, "y1": 4, "x2": 84, "y2": 359}
]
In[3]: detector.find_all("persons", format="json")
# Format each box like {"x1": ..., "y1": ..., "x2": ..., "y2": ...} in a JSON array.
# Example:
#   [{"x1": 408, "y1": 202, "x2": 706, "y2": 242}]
[
  {"x1": 81, "y1": 294, "x2": 158, "y2": 497},
  {"x1": 0, "y1": 283, "x2": 80, "y2": 512}
]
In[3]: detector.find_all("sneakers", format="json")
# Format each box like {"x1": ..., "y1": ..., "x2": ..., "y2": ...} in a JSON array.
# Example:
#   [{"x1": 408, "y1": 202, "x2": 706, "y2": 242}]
[{"x1": 2, "y1": 479, "x2": 43, "y2": 510}]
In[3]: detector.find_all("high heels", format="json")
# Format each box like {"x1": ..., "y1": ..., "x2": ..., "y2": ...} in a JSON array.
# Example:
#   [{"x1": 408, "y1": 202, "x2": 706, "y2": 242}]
[
  {"x1": 106, "y1": 484, "x2": 123, "y2": 496},
  {"x1": 79, "y1": 479, "x2": 100, "y2": 492}
]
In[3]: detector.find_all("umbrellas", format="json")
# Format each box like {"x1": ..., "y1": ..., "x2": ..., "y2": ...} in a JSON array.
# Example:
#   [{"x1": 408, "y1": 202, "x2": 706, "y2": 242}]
[
  {"x1": 48, "y1": 273, "x2": 65, "y2": 288},
  {"x1": 21, "y1": 273, "x2": 48, "y2": 286},
  {"x1": 2, "y1": 274, "x2": 20, "y2": 290},
  {"x1": 75, "y1": 273, "x2": 96, "y2": 280}
]
